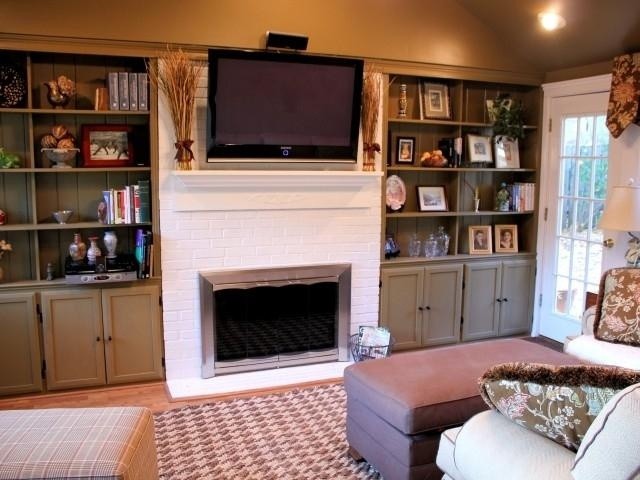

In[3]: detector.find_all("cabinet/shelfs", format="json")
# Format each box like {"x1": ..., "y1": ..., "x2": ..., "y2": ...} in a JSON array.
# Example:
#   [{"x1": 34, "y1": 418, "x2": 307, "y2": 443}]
[
  {"x1": 380, "y1": 57, "x2": 547, "y2": 267},
  {"x1": 0, "y1": 41, "x2": 160, "y2": 289},
  {"x1": 377, "y1": 257, "x2": 465, "y2": 355},
  {"x1": 39, "y1": 279, "x2": 166, "y2": 394},
  {"x1": 461, "y1": 251, "x2": 539, "y2": 344},
  {"x1": 0, "y1": 286, "x2": 45, "y2": 398}
]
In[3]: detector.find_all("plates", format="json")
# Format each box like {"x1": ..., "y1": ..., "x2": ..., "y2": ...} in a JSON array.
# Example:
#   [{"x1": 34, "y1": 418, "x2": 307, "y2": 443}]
[{"x1": 385, "y1": 175, "x2": 407, "y2": 212}]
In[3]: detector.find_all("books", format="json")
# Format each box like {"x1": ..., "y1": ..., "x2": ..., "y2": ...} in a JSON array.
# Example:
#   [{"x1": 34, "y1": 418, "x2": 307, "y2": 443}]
[
  {"x1": 108, "y1": 72, "x2": 148, "y2": 112},
  {"x1": 492, "y1": 182, "x2": 535, "y2": 213},
  {"x1": 102, "y1": 180, "x2": 154, "y2": 279}
]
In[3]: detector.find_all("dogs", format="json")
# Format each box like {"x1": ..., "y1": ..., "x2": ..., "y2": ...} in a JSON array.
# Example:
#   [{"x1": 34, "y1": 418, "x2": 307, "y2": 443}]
[{"x1": 91, "y1": 138, "x2": 128, "y2": 160}]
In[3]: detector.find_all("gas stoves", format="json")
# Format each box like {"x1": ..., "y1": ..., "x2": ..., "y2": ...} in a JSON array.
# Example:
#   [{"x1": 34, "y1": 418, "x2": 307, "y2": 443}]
[{"x1": 64, "y1": 253, "x2": 138, "y2": 284}]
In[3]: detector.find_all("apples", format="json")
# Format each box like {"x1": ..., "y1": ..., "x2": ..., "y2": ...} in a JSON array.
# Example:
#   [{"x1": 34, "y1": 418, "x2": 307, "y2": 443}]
[{"x1": 420, "y1": 150, "x2": 443, "y2": 162}]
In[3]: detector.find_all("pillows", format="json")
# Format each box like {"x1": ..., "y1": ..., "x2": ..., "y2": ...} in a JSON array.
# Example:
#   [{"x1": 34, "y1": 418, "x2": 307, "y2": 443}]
[
  {"x1": 591, "y1": 262, "x2": 640, "y2": 349},
  {"x1": 474, "y1": 360, "x2": 640, "y2": 456}
]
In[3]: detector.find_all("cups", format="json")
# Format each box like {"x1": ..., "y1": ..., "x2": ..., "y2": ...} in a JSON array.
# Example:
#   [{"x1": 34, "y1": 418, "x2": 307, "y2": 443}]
[
  {"x1": 103, "y1": 230, "x2": 118, "y2": 259},
  {"x1": 472, "y1": 199, "x2": 481, "y2": 213},
  {"x1": 93, "y1": 87, "x2": 109, "y2": 111}
]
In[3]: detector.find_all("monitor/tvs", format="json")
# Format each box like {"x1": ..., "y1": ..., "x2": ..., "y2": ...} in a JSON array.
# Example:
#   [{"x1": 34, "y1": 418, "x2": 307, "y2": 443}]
[{"x1": 206, "y1": 47, "x2": 365, "y2": 160}]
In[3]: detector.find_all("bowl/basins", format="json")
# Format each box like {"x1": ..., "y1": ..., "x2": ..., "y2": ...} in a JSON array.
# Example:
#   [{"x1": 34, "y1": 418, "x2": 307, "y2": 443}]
[
  {"x1": 50, "y1": 210, "x2": 73, "y2": 224},
  {"x1": 420, "y1": 160, "x2": 449, "y2": 168}
]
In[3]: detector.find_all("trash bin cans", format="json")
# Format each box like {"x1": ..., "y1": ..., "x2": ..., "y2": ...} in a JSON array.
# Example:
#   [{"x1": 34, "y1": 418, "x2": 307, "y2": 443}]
[{"x1": 348, "y1": 333, "x2": 396, "y2": 362}]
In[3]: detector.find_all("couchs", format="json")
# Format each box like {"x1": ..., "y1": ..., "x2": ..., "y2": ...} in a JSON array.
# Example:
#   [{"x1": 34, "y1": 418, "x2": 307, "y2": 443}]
[{"x1": 432, "y1": 301, "x2": 640, "y2": 478}]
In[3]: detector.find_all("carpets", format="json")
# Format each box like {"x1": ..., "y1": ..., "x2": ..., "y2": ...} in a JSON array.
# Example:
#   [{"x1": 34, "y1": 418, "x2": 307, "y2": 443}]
[
  {"x1": 520, "y1": 332, "x2": 567, "y2": 354},
  {"x1": 152, "y1": 378, "x2": 385, "y2": 479}
]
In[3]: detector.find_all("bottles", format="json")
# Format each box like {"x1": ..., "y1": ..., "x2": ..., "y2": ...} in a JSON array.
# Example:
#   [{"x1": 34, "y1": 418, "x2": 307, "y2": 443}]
[
  {"x1": 406, "y1": 232, "x2": 422, "y2": 258},
  {"x1": 422, "y1": 226, "x2": 452, "y2": 260},
  {"x1": 45, "y1": 261, "x2": 57, "y2": 281},
  {"x1": 496, "y1": 182, "x2": 510, "y2": 212},
  {"x1": 69, "y1": 233, "x2": 103, "y2": 263}
]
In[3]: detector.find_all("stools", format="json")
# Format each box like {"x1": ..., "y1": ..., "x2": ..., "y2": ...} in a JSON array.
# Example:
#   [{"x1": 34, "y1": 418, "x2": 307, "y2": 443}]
[
  {"x1": 330, "y1": 330, "x2": 588, "y2": 479},
  {"x1": 0, "y1": 405, "x2": 165, "y2": 480}
]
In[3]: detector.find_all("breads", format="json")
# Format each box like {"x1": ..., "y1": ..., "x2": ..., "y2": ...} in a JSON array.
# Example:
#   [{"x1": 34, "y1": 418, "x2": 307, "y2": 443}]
[{"x1": 40, "y1": 123, "x2": 76, "y2": 149}]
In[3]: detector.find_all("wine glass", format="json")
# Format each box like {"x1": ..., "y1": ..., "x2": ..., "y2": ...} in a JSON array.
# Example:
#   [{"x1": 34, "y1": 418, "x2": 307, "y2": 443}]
[{"x1": 42, "y1": 148, "x2": 79, "y2": 168}]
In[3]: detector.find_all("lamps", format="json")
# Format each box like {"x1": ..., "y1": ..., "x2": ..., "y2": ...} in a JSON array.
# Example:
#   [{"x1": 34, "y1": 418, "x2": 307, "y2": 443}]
[{"x1": 592, "y1": 175, "x2": 640, "y2": 267}]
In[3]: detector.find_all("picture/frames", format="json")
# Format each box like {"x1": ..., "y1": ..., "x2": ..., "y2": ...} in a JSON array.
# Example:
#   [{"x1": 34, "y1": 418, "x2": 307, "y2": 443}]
[
  {"x1": 492, "y1": 134, "x2": 523, "y2": 169},
  {"x1": 414, "y1": 183, "x2": 451, "y2": 213},
  {"x1": 465, "y1": 132, "x2": 495, "y2": 165},
  {"x1": 465, "y1": 222, "x2": 494, "y2": 257},
  {"x1": 493, "y1": 223, "x2": 519, "y2": 254},
  {"x1": 395, "y1": 134, "x2": 417, "y2": 166},
  {"x1": 79, "y1": 121, "x2": 137, "y2": 168},
  {"x1": 417, "y1": 78, "x2": 453, "y2": 121}
]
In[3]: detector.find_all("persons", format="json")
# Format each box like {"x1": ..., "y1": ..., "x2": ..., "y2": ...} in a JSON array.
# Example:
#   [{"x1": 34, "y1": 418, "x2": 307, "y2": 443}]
[
  {"x1": 474, "y1": 230, "x2": 487, "y2": 250},
  {"x1": 500, "y1": 229, "x2": 513, "y2": 249},
  {"x1": 92, "y1": 139, "x2": 129, "y2": 159}
]
